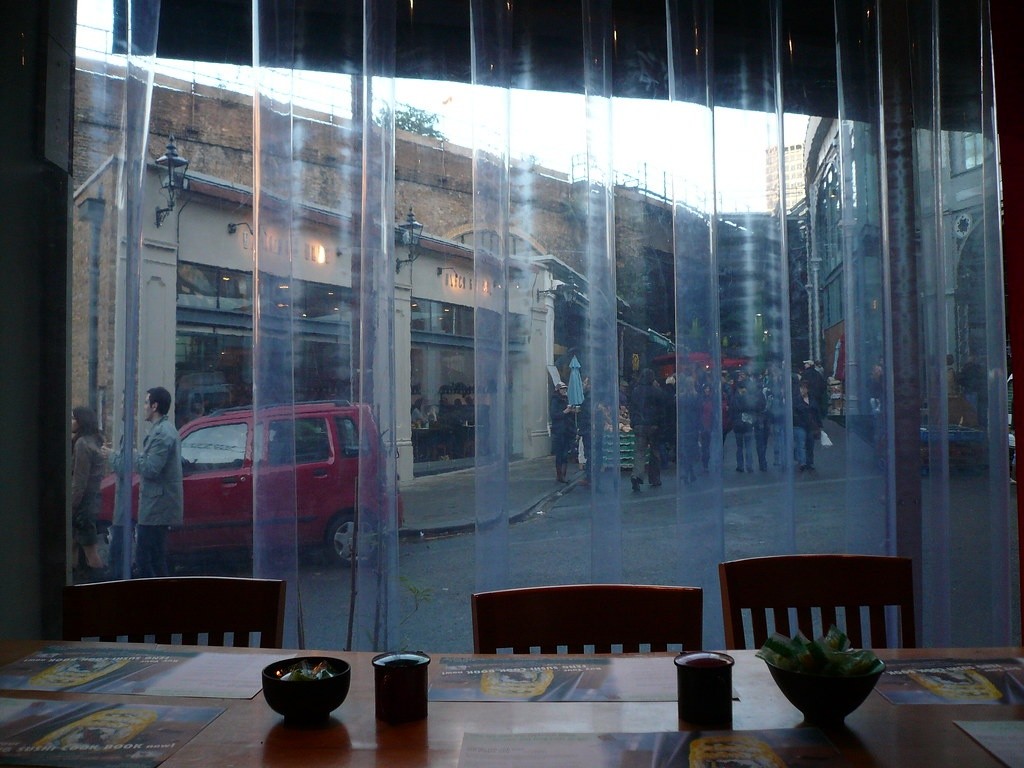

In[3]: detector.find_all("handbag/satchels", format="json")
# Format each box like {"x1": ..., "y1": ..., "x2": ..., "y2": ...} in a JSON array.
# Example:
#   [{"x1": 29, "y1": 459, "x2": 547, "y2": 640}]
[
  {"x1": 821, "y1": 430, "x2": 834, "y2": 449},
  {"x1": 742, "y1": 412, "x2": 754, "y2": 425}
]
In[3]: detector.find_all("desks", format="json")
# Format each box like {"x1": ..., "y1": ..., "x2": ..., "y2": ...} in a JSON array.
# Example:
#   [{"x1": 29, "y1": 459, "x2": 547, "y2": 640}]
[{"x1": 0, "y1": 644, "x2": 1024, "y2": 768}]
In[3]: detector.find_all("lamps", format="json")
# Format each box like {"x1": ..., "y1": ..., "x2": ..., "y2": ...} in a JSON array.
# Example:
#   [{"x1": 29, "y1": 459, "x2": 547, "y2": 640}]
[
  {"x1": 228, "y1": 222, "x2": 253, "y2": 237},
  {"x1": 154, "y1": 134, "x2": 190, "y2": 228},
  {"x1": 395, "y1": 205, "x2": 423, "y2": 274},
  {"x1": 437, "y1": 267, "x2": 459, "y2": 278}
]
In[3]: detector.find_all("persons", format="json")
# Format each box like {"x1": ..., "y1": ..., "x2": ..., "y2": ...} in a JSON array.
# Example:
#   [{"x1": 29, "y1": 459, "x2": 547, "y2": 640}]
[
  {"x1": 70, "y1": 406, "x2": 104, "y2": 584},
  {"x1": 411, "y1": 398, "x2": 473, "y2": 430},
  {"x1": 578, "y1": 360, "x2": 829, "y2": 492},
  {"x1": 101, "y1": 386, "x2": 184, "y2": 578},
  {"x1": 549, "y1": 382, "x2": 578, "y2": 483}
]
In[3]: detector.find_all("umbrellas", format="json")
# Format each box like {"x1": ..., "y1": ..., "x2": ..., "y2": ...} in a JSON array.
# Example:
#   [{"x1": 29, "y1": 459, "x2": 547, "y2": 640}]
[{"x1": 567, "y1": 354, "x2": 584, "y2": 426}]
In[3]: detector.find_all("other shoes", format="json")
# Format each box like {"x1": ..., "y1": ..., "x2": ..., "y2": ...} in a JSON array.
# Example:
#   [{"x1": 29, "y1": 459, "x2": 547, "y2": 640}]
[
  {"x1": 631, "y1": 476, "x2": 641, "y2": 490},
  {"x1": 735, "y1": 468, "x2": 744, "y2": 472},
  {"x1": 649, "y1": 480, "x2": 662, "y2": 485},
  {"x1": 579, "y1": 480, "x2": 588, "y2": 485},
  {"x1": 800, "y1": 465, "x2": 807, "y2": 471},
  {"x1": 809, "y1": 464, "x2": 815, "y2": 469}
]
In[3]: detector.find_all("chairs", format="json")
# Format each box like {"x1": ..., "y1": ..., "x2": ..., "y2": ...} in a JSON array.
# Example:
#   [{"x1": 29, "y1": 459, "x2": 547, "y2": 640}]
[
  {"x1": 719, "y1": 554, "x2": 917, "y2": 651},
  {"x1": 64, "y1": 576, "x2": 285, "y2": 651},
  {"x1": 471, "y1": 583, "x2": 705, "y2": 654}
]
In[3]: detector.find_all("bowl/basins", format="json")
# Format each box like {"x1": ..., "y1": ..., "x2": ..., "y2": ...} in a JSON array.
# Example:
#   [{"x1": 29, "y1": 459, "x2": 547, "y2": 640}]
[
  {"x1": 262, "y1": 658, "x2": 351, "y2": 731},
  {"x1": 764, "y1": 657, "x2": 886, "y2": 727}
]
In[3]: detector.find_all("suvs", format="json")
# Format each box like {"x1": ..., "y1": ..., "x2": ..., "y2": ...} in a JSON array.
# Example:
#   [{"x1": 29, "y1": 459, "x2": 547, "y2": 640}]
[{"x1": 99, "y1": 399, "x2": 404, "y2": 569}]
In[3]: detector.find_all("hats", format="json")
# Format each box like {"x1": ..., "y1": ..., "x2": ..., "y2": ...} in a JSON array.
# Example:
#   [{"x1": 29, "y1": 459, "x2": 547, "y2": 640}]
[
  {"x1": 803, "y1": 360, "x2": 814, "y2": 365},
  {"x1": 556, "y1": 381, "x2": 567, "y2": 389}
]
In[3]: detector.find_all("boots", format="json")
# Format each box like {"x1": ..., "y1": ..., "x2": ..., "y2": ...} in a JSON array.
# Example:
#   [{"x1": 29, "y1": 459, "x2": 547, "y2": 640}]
[
  {"x1": 557, "y1": 466, "x2": 560, "y2": 480},
  {"x1": 560, "y1": 464, "x2": 568, "y2": 482}
]
